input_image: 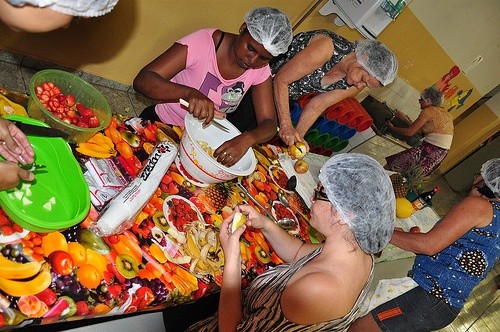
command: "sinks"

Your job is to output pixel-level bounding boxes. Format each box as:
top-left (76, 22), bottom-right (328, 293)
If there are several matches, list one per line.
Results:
top-left (360, 94), bottom-right (419, 148)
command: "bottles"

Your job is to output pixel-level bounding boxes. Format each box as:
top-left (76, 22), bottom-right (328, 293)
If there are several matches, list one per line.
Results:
top-left (411, 186), bottom-right (441, 211)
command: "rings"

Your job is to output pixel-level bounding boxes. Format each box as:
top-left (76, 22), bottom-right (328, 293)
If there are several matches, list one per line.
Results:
top-left (222, 150), bottom-right (228, 156)
top-left (229, 155), bottom-right (234, 160)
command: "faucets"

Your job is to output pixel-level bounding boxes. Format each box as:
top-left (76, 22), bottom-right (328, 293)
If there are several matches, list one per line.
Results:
top-left (383, 102), bottom-right (401, 121)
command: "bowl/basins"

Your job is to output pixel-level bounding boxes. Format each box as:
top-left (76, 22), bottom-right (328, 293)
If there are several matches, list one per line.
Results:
top-left (0, 115), bottom-right (90, 231)
top-left (27, 68), bottom-right (112, 143)
top-left (173, 111), bottom-right (257, 188)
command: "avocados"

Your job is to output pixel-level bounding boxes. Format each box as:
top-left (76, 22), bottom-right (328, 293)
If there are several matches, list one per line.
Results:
top-left (77, 227), bottom-right (112, 254)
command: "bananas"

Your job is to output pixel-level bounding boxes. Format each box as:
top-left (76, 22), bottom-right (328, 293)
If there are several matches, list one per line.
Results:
top-left (75, 132), bottom-right (117, 158)
top-left (232, 212), bottom-right (246, 233)
top-left (0, 250), bottom-right (52, 296)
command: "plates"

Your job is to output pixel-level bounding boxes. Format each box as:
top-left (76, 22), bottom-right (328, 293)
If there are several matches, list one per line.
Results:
top-left (164, 195), bottom-right (206, 237)
top-left (271, 201), bottom-right (300, 234)
top-left (269, 165), bottom-right (297, 193)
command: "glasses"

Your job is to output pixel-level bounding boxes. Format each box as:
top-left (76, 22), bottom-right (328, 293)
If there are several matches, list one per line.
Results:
top-left (313, 188), bottom-right (331, 202)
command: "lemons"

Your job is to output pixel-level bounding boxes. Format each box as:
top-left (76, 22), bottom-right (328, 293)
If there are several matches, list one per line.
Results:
top-left (93, 304), bottom-right (110, 316)
top-left (150, 244), bottom-right (168, 263)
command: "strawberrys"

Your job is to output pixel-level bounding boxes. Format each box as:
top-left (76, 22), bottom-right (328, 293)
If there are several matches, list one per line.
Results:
top-left (0, 207), bottom-right (23, 236)
top-left (272, 203), bottom-right (298, 231)
top-left (36, 81), bottom-right (99, 128)
top-left (166, 197), bottom-right (198, 232)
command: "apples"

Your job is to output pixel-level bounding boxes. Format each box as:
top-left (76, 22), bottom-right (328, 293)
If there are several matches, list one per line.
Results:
top-left (244, 166), bottom-right (277, 213)
top-left (103, 115), bottom-right (159, 178)
top-left (409, 226), bottom-right (421, 234)
top-left (294, 160), bottom-right (309, 174)
top-left (76, 301), bottom-right (90, 316)
top-left (291, 142), bottom-right (307, 158)
top-left (40, 232), bottom-right (106, 288)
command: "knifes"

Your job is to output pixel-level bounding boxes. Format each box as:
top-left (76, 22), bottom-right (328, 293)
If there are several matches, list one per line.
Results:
top-left (180, 99), bottom-right (228, 130)
top-left (20, 165), bottom-right (45, 171)
top-left (277, 127), bottom-right (302, 153)
top-left (5, 119), bottom-right (70, 137)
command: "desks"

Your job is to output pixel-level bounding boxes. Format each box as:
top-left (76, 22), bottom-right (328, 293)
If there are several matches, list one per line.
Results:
top-left (0, 87), bottom-right (443, 330)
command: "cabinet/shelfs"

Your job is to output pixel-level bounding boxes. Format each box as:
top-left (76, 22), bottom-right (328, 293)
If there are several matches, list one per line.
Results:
top-left (318, 0), bottom-right (413, 40)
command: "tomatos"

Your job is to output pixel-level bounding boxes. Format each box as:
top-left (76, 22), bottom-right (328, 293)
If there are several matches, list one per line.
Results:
top-left (19, 232), bottom-right (48, 262)
top-left (160, 175), bottom-right (178, 194)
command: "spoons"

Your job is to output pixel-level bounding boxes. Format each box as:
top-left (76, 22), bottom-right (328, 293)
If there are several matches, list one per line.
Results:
top-left (236, 183), bottom-right (296, 228)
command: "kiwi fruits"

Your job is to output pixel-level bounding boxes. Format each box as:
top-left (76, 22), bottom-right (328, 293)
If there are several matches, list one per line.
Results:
top-left (255, 246), bottom-right (271, 263)
top-left (116, 254), bottom-right (138, 278)
top-left (153, 211), bottom-right (171, 232)
top-left (234, 189), bottom-right (249, 202)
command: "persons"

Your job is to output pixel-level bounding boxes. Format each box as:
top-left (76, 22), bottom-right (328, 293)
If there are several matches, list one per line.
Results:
top-left (133, 6), bottom-right (293, 168)
top-left (346, 157), bottom-right (500, 332)
top-left (229, 29), bottom-right (399, 161)
top-left (0, 0), bottom-right (119, 192)
top-left (184, 153), bottom-right (396, 332)
top-left (382, 88), bottom-right (454, 179)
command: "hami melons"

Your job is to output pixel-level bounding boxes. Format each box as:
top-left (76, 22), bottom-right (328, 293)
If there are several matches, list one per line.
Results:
top-left (395, 198), bottom-right (414, 218)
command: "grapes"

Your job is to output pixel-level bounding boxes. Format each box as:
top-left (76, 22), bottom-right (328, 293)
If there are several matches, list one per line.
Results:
top-left (52, 270), bottom-right (169, 307)
top-left (173, 180), bottom-right (193, 200)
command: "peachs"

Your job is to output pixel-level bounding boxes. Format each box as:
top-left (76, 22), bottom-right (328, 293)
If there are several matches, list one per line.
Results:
top-left (18, 296), bottom-right (48, 318)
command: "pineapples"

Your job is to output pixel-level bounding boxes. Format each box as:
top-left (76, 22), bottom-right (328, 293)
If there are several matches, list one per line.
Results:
top-left (206, 183), bottom-right (234, 212)
top-left (390, 162), bottom-right (423, 198)
